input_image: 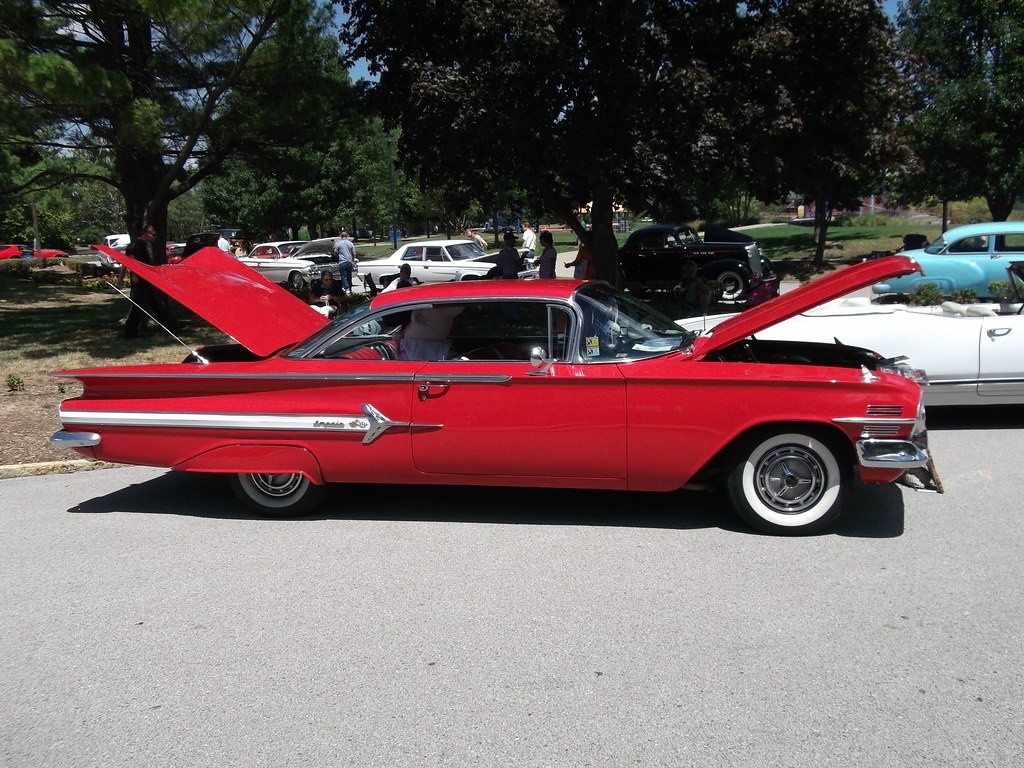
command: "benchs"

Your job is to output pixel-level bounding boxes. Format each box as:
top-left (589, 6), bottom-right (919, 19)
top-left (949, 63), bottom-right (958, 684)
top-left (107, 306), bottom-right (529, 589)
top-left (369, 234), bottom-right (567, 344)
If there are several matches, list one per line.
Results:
top-left (330, 337), bottom-right (407, 361)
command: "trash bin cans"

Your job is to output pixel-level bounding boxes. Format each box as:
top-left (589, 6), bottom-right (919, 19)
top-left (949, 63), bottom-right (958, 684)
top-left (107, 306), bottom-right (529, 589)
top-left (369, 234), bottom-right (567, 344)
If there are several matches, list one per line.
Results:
top-left (389, 229), bottom-right (402, 243)
top-left (903, 234), bottom-right (927, 251)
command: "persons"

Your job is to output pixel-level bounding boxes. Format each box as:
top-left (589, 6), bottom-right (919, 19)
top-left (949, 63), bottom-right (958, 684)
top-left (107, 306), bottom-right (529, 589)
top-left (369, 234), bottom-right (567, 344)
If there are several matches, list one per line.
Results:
top-left (278, 224), bottom-right (311, 242)
top-left (497, 232), bottom-right (527, 279)
top-left (466, 228), bottom-right (488, 250)
top-left (575, 214), bottom-right (586, 247)
top-left (516, 217), bottom-right (521, 234)
top-left (218, 232), bottom-right (255, 257)
top-left (117, 219), bottom-right (157, 339)
top-left (309, 270), bottom-right (345, 317)
top-left (521, 222), bottom-right (536, 258)
top-left (533, 231), bottom-right (558, 279)
top-left (564, 229), bottom-right (598, 279)
top-left (485, 220), bottom-right (489, 229)
top-left (45, 238), bottom-right (51, 247)
top-left (334, 232), bottom-right (357, 294)
top-left (4, 233), bottom-right (33, 247)
top-left (368, 263), bottom-right (417, 296)
top-left (382, 280), bottom-right (412, 325)
top-left (401, 227), bottom-right (406, 236)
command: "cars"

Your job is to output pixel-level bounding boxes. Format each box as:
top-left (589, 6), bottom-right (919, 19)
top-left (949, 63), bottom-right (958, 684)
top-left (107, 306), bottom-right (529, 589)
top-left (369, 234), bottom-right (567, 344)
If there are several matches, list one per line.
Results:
top-left (0, 244), bottom-right (69, 259)
top-left (616, 223), bottom-right (781, 306)
top-left (356, 240), bottom-right (540, 287)
top-left (166, 229), bottom-right (359, 295)
top-left (50, 245), bottom-right (945, 537)
top-left (672, 221), bottom-right (1024, 405)
top-left (98, 234), bottom-right (131, 269)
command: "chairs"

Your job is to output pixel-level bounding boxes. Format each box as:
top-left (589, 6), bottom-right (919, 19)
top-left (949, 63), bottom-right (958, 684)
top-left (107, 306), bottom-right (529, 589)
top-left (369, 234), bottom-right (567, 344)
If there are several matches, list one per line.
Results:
top-left (733, 275), bottom-right (781, 311)
top-left (453, 343), bottom-right (527, 360)
top-left (895, 234), bottom-right (930, 254)
top-left (364, 273), bottom-right (402, 299)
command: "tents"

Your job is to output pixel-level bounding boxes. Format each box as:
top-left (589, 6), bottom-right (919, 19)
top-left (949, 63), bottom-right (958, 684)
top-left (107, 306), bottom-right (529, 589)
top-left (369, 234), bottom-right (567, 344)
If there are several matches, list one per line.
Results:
top-left (573, 196), bottom-right (628, 233)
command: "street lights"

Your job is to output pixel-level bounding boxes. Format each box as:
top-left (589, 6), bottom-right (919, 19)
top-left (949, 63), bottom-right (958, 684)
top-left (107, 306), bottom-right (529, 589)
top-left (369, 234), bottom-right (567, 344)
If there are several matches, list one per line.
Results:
top-left (384, 131), bottom-right (397, 250)
